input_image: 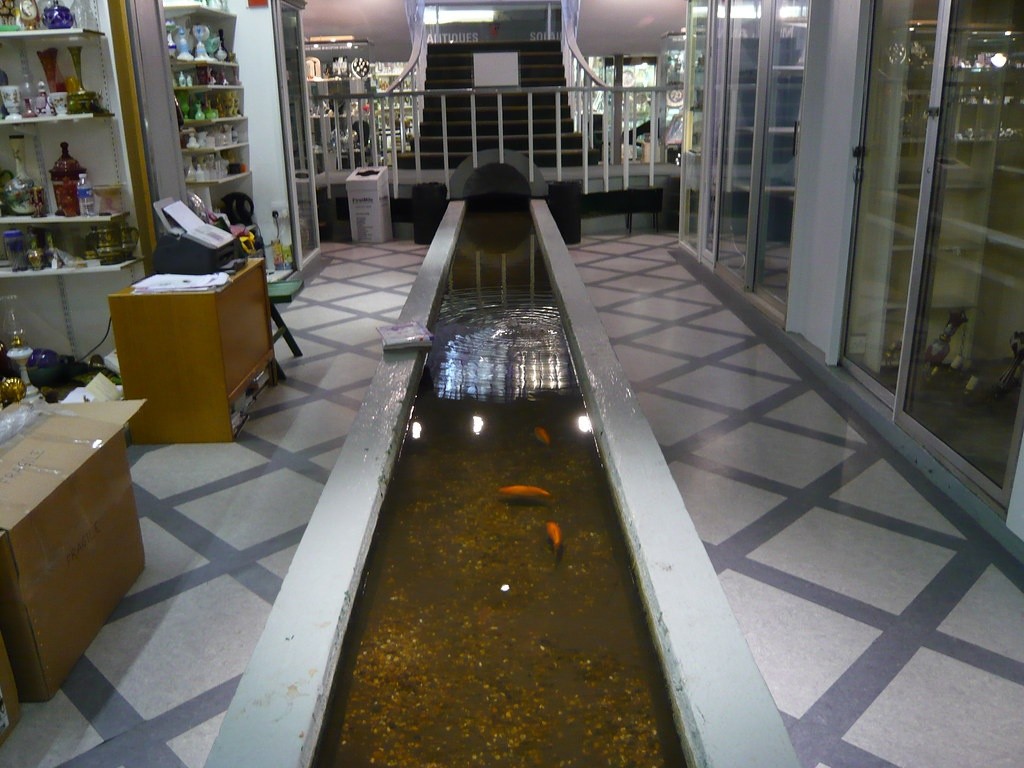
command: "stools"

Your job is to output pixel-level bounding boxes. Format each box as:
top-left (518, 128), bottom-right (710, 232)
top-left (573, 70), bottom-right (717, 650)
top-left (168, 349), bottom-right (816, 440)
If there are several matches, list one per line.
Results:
top-left (266, 280), bottom-right (304, 358)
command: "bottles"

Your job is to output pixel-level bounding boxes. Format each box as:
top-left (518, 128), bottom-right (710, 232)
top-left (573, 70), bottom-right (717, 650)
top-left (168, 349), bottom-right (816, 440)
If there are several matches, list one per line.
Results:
top-left (76, 173), bottom-right (94, 217)
top-left (179, 70), bottom-right (192, 87)
top-left (23, 98), bottom-right (37, 118)
top-left (3, 228), bottom-right (27, 272)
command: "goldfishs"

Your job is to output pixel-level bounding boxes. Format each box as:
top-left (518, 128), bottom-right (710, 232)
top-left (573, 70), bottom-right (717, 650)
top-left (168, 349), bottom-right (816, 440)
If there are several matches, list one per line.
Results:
top-left (544, 520), bottom-right (562, 554)
top-left (497, 486), bottom-right (549, 500)
top-left (534, 425), bottom-right (550, 444)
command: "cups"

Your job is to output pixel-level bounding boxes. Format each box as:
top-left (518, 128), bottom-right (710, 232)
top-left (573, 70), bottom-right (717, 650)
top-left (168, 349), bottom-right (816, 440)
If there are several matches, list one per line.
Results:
top-left (0, 85), bottom-right (23, 120)
top-left (49, 92), bottom-right (68, 117)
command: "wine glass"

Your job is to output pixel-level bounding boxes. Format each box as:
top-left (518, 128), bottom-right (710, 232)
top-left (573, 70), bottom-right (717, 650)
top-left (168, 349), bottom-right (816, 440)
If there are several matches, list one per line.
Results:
top-left (28, 186), bottom-right (47, 218)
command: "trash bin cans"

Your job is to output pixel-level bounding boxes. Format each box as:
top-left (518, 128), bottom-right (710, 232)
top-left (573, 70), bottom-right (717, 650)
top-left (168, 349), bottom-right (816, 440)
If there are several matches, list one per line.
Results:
top-left (295, 170), bottom-right (315, 249)
top-left (345, 165), bottom-right (394, 244)
top-left (411, 181), bottom-right (448, 245)
top-left (547, 180), bottom-right (581, 246)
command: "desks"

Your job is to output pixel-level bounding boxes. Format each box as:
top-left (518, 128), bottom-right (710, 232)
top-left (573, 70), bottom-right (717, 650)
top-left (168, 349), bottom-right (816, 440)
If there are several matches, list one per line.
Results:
top-left (107, 257), bottom-right (278, 444)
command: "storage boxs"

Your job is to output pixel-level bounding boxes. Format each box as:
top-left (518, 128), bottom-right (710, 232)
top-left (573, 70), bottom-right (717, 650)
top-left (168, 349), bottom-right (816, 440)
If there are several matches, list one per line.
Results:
top-left (0, 399), bottom-right (151, 702)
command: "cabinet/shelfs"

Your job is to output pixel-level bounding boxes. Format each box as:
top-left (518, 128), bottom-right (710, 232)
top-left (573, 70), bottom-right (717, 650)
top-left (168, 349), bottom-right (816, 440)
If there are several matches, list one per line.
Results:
top-left (0, 29), bottom-right (139, 274)
top-left (864, 0), bottom-right (1015, 372)
top-left (305, 40), bottom-right (370, 156)
top-left (169, 55), bottom-right (252, 184)
